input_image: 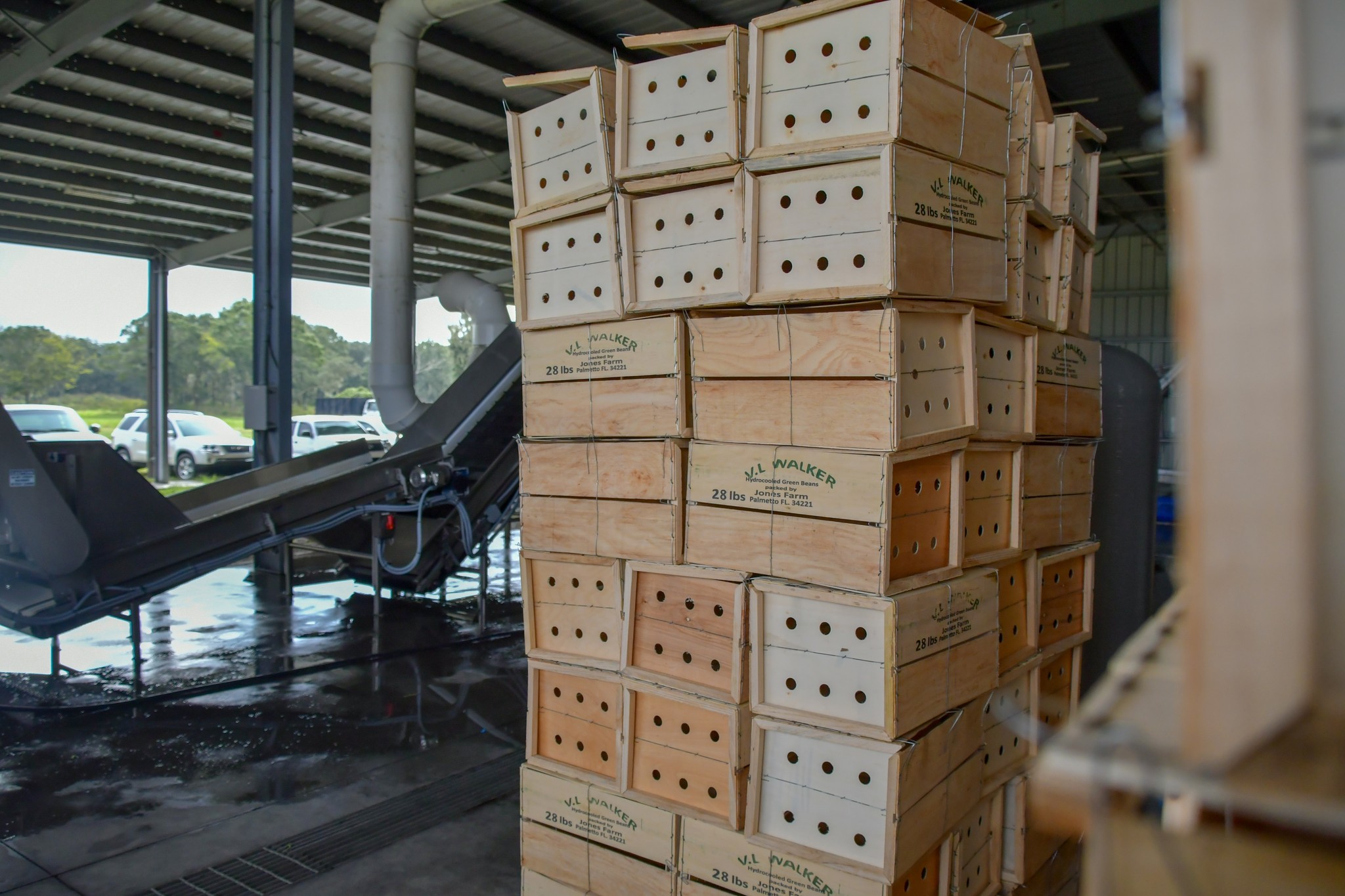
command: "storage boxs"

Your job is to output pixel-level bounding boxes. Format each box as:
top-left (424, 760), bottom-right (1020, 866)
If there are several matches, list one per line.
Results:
top-left (496, 0), bottom-right (1107, 896)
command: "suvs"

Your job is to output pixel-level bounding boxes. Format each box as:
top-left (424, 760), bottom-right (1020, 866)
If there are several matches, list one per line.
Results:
top-left (110, 409), bottom-right (255, 482)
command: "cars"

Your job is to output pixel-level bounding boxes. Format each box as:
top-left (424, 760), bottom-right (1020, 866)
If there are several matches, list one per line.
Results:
top-left (288, 400), bottom-right (400, 462)
top-left (1, 403), bottom-right (114, 447)
